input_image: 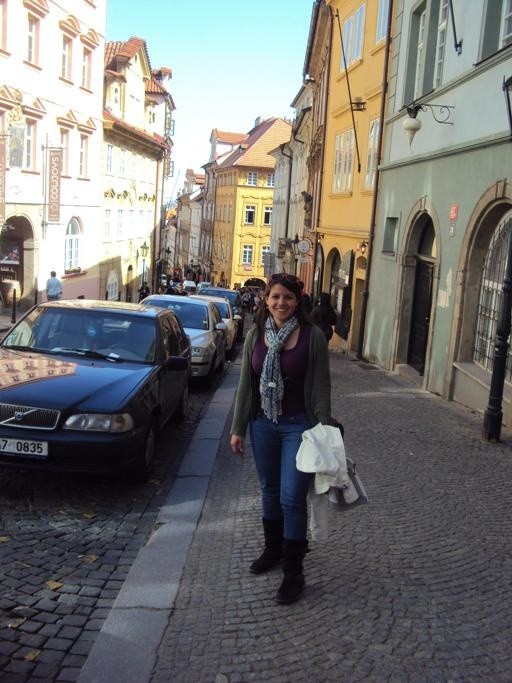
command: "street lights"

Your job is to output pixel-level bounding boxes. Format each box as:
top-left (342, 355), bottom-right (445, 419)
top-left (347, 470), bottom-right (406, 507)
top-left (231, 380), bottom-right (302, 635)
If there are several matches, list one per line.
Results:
top-left (163, 246), bottom-right (172, 279)
top-left (139, 242), bottom-right (150, 290)
top-left (477, 73), bottom-right (512, 443)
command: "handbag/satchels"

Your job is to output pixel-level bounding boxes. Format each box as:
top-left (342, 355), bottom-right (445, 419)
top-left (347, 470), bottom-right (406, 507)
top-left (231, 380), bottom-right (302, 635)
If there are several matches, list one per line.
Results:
top-left (322, 323), bottom-right (333, 340)
top-left (323, 452), bottom-right (369, 512)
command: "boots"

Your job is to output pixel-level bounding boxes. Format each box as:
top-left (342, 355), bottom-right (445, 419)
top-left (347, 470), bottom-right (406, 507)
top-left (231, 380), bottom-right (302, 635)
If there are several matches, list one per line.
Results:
top-left (249, 517), bottom-right (284, 575)
top-left (275, 534), bottom-right (309, 604)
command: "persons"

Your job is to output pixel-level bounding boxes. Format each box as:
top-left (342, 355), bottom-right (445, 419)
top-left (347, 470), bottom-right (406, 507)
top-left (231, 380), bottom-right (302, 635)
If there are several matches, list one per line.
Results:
top-left (137, 263), bottom-right (265, 315)
top-left (300, 281), bottom-right (313, 313)
top-left (46, 271), bottom-right (64, 302)
top-left (229, 272), bottom-right (336, 604)
top-left (310, 292), bottom-right (338, 346)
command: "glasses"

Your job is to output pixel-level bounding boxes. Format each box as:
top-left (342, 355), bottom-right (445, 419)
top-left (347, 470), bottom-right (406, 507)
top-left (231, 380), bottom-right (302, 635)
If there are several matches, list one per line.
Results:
top-left (268, 274), bottom-right (301, 289)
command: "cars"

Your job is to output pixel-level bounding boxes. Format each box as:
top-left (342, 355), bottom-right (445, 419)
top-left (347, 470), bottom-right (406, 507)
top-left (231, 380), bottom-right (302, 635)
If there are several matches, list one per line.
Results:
top-left (1, 294), bottom-right (192, 495)
top-left (139, 272), bottom-right (261, 385)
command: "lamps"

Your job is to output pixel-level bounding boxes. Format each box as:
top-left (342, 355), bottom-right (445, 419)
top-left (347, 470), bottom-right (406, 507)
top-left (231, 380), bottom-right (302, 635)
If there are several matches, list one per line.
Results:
top-left (360, 240), bottom-right (368, 256)
top-left (290, 234), bottom-right (313, 256)
top-left (403, 101), bottom-right (455, 149)
